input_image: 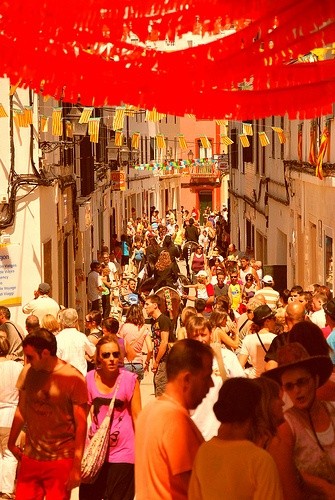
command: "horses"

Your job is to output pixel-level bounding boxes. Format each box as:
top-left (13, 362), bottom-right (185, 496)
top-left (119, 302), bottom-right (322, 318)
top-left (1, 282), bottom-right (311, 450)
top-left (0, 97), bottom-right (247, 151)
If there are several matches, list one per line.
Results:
top-left (182, 240), bottom-right (198, 282)
top-left (134, 273), bottom-right (190, 331)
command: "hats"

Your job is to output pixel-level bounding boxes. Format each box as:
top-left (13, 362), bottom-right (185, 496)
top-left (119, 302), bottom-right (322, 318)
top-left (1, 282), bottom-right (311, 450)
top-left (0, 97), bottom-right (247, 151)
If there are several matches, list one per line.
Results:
top-left (322, 297), bottom-right (335, 322)
top-left (39, 283), bottom-right (51, 294)
top-left (260, 343), bottom-right (333, 390)
top-left (214, 378), bottom-right (260, 423)
top-left (227, 255), bottom-right (238, 262)
top-left (252, 305), bottom-right (276, 322)
top-left (261, 275), bottom-right (273, 284)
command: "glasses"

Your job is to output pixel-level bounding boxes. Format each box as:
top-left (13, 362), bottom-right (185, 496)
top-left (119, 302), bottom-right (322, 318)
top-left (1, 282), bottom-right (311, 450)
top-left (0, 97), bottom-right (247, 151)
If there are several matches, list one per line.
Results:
top-left (246, 278), bottom-right (252, 282)
top-left (231, 276), bottom-right (238, 279)
top-left (269, 317), bottom-right (276, 322)
top-left (85, 319), bottom-right (91, 323)
top-left (283, 376), bottom-right (312, 391)
top-left (101, 351), bottom-right (120, 358)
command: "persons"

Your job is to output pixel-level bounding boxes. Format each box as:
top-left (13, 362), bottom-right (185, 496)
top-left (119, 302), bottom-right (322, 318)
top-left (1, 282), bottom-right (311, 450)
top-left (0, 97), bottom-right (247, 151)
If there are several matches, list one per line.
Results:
top-left (85, 205), bottom-right (335, 442)
top-left (56, 308), bottom-right (96, 378)
top-left (135, 339), bottom-right (215, 500)
top-left (26, 315), bottom-right (39, 334)
top-left (79, 336), bottom-right (141, 500)
top-left (8, 328), bottom-right (86, 500)
top-left (187, 377), bottom-right (284, 500)
top-left (40, 314), bottom-right (60, 335)
top-left (22, 283), bottom-right (60, 324)
top-left (0, 336), bottom-right (24, 499)
top-left (0, 306), bottom-right (25, 366)
top-left (260, 343), bottom-right (335, 500)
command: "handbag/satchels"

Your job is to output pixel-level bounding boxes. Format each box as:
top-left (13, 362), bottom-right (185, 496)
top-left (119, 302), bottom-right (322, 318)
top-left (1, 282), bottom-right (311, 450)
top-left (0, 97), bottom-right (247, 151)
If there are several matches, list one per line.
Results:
top-left (78, 370), bottom-right (127, 485)
